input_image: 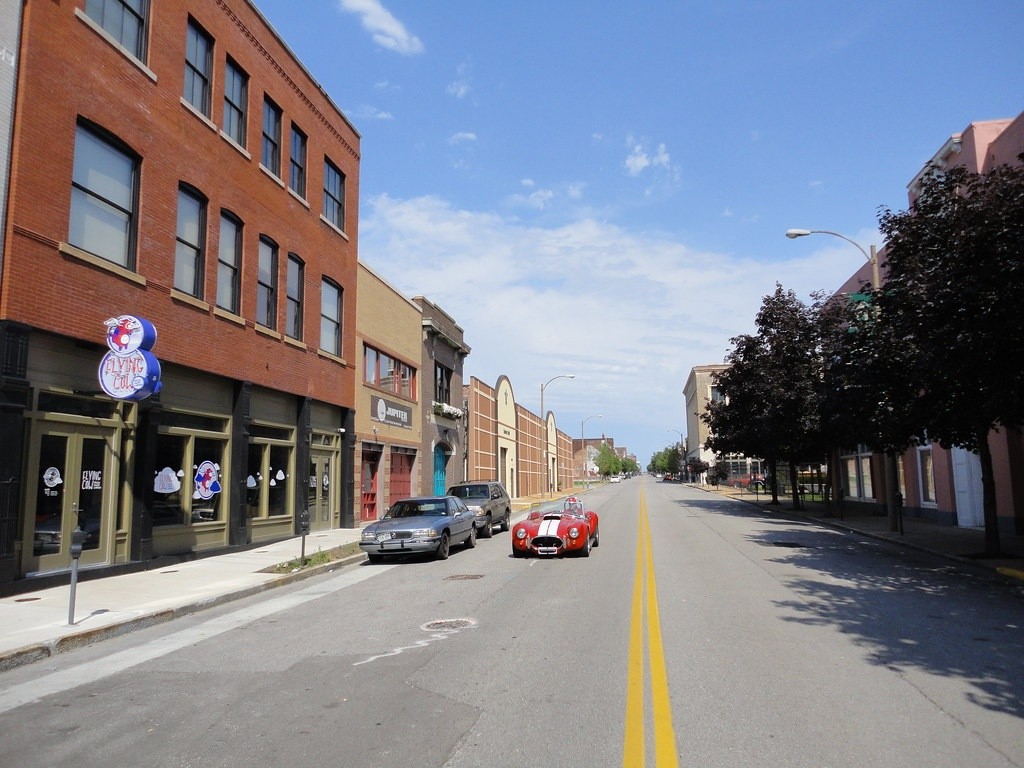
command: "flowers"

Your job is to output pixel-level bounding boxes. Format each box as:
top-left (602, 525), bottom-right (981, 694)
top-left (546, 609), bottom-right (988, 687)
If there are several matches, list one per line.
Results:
top-left (434, 402), bottom-right (462, 418)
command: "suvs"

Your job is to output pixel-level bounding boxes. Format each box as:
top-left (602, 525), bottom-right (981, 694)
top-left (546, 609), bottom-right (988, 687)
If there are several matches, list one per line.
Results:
top-left (435, 478), bottom-right (512, 538)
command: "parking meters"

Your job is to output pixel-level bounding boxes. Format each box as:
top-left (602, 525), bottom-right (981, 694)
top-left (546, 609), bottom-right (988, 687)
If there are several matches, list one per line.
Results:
top-left (838, 488), bottom-right (845, 522)
top-left (301, 510), bottom-right (311, 564)
top-left (68, 526), bottom-right (85, 627)
top-left (896, 492), bottom-right (904, 532)
top-left (550, 484), bottom-right (553, 498)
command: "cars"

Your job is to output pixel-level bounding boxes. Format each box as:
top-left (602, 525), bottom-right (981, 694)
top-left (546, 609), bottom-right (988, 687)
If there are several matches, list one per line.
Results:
top-left (610, 476), bottom-right (620, 483)
top-left (620, 473), bottom-right (632, 480)
top-left (192, 504), bottom-right (215, 523)
top-left (33, 513), bottom-right (102, 551)
top-left (650, 472), bottom-right (672, 483)
top-left (358, 495), bottom-right (476, 563)
top-left (748, 478), bottom-right (765, 489)
top-left (727, 472), bottom-right (762, 489)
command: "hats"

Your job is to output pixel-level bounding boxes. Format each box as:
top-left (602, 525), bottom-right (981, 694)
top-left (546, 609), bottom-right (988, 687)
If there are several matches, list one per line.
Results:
top-left (568, 498), bottom-right (576, 504)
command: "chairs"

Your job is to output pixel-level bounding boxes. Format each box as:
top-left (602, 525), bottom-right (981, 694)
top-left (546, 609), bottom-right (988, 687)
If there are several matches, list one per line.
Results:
top-left (564, 497), bottom-right (582, 511)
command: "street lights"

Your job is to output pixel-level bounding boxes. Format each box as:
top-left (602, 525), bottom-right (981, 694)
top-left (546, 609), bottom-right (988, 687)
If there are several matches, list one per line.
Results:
top-left (668, 430), bottom-right (684, 447)
top-left (541, 375), bottom-right (575, 498)
top-left (582, 415), bottom-right (602, 489)
top-left (662, 439), bottom-right (674, 449)
top-left (786, 228), bottom-right (902, 532)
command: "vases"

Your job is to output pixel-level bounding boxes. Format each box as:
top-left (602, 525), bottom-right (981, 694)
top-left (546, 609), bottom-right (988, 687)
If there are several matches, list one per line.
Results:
top-left (435, 412), bottom-right (461, 420)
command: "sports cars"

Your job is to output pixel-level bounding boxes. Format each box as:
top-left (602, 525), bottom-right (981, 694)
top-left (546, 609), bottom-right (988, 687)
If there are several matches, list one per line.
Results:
top-left (512, 497), bottom-right (599, 559)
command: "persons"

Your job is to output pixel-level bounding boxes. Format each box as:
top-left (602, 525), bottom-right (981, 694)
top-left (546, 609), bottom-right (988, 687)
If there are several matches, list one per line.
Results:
top-left (563, 498), bottom-right (587, 518)
